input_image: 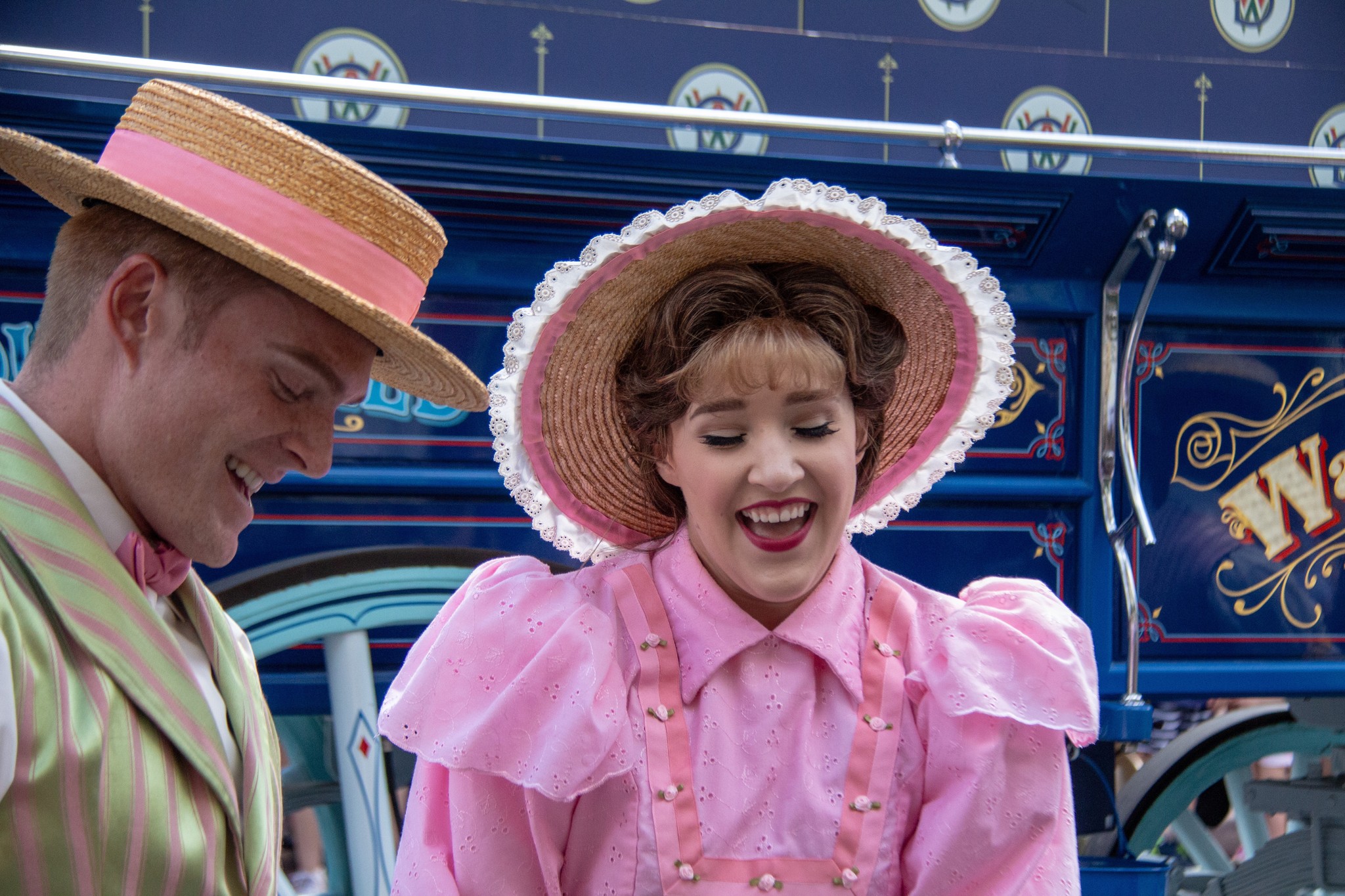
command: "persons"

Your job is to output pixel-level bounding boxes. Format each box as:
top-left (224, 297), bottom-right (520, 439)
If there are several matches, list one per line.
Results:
top-left (277, 738), bottom-right (328, 896)
top-left (375, 178), bottom-right (1098, 896)
top-left (1136, 697), bottom-right (1292, 896)
top-left (0, 80), bottom-right (489, 896)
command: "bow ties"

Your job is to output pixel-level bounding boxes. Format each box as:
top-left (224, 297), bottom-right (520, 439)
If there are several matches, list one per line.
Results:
top-left (114, 532), bottom-right (192, 598)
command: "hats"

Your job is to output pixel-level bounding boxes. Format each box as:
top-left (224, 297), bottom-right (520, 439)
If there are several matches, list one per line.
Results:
top-left (0, 77), bottom-right (489, 413)
top-left (488, 177), bottom-right (1017, 564)
